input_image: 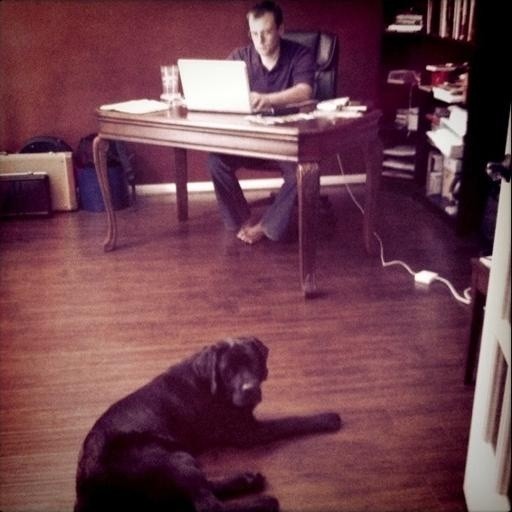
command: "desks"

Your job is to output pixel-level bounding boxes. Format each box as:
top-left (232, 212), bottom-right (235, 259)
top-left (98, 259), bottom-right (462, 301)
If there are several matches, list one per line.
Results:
top-left (92, 99), bottom-right (384, 299)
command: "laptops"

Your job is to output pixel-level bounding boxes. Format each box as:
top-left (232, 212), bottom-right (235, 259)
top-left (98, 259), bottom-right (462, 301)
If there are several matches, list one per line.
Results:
top-left (177, 58), bottom-right (264, 114)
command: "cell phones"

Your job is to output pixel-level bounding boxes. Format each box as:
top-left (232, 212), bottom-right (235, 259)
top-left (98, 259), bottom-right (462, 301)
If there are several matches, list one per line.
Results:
top-left (259, 105), bottom-right (300, 118)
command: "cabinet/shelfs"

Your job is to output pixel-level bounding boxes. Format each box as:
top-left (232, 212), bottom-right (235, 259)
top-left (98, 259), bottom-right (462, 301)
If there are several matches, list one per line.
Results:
top-left (378, 1), bottom-right (512, 238)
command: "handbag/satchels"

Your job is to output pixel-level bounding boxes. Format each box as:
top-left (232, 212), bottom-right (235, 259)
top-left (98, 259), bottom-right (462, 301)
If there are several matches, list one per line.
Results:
top-left (76, 134), bottom-right (120, 167)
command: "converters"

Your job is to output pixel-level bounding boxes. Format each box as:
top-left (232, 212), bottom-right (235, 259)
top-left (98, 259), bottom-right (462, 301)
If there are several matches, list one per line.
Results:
top-left (414, 269), bottom-right (439, 285)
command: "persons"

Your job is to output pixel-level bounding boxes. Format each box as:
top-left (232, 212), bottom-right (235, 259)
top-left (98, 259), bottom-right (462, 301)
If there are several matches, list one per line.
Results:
top-left (205, 3), bottom-right (315, 246)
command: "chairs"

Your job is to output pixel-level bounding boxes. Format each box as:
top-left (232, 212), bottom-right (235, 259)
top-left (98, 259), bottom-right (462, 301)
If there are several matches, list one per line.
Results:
top-left (242, 31), bottom-right (340, 213)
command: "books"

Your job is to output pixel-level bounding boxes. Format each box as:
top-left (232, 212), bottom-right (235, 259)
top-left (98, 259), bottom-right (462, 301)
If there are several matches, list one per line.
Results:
top-left (383, 1), bottom-right (476, 183)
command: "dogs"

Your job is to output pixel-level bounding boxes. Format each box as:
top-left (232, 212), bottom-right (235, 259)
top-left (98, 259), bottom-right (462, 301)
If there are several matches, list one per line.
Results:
top-left (72, 334), bottom-right (344, 511)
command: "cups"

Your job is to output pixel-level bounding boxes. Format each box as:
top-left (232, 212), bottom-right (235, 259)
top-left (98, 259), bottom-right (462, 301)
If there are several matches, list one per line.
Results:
top-left (160, 65), bottom-right (179, 101)
